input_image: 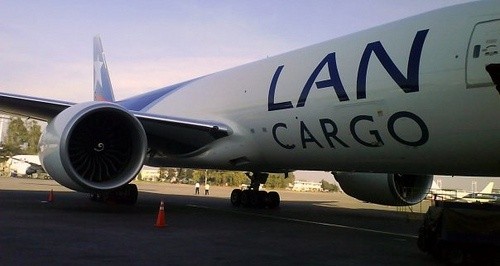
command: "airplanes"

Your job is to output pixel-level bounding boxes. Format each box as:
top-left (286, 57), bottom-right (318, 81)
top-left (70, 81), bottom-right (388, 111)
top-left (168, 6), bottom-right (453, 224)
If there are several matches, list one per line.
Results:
top-left (0, 0), bottom-right (500, 206)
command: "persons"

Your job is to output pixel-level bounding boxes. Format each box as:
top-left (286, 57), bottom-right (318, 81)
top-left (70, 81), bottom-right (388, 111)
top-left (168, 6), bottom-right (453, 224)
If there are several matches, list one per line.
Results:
top-left (194, 181), bottom-right (200, 195)
top-left (204, 182), bottom-right (210, 196)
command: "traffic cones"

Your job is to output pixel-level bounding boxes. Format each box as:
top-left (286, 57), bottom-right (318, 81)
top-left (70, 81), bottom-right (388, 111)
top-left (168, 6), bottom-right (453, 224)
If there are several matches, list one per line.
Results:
top-left (48, 188), bottom-right (54, 201)
top-left (153, 200), bottom-right (169, 227)
